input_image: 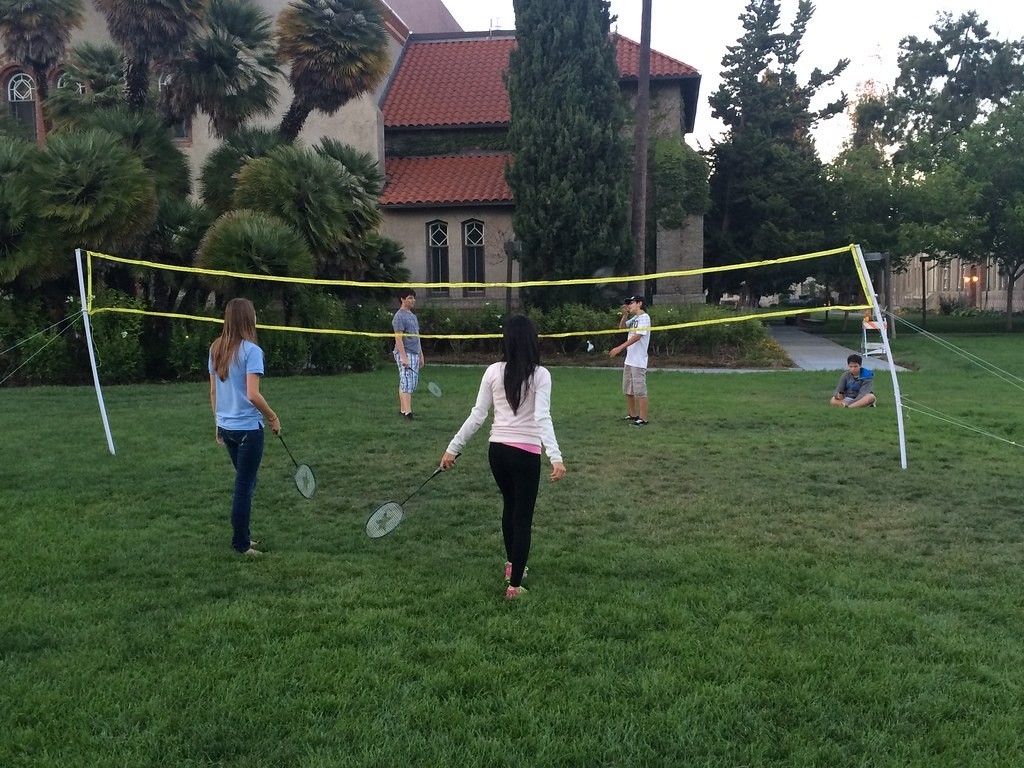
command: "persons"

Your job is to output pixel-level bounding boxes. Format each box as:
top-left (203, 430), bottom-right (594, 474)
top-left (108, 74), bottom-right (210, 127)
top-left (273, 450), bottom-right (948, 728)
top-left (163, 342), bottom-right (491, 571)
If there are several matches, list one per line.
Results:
top-left (440, 315), bottom-right (566, 601)
top-left (830, 354), bottom-right (877, 408)
top-left (208, 297), bottom-right (281, 555)
top-left (391, 288), bottom-right (424, 420)
top-left (608, 296), bottom-right (651, 426)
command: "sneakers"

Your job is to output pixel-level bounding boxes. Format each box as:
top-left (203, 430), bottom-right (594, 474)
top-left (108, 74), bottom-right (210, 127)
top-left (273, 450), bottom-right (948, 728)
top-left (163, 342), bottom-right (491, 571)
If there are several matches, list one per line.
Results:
top-left (507, 584), bottom-right (529, 598)
top-left (623, 415), bottom-right (640, 420)
top-left (632, 419), bottom-right (648, 427)
top-left (504, 561), bottom-right (529, 581)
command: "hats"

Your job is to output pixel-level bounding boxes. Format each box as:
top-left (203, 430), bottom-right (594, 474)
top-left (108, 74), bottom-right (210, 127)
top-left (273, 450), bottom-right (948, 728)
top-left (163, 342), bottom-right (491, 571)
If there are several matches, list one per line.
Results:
top-left (624, 295), bottom-right (646, 305)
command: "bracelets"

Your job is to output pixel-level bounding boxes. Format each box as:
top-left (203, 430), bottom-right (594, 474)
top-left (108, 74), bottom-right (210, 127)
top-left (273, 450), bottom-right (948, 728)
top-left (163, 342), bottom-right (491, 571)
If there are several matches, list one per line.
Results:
top-left (269, 417), bottom-right (278, 422)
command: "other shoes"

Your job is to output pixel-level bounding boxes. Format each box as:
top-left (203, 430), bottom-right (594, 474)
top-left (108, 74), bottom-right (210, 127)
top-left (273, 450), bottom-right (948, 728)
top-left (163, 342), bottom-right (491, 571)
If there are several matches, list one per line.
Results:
top-left (405, 412), bottom-right (413, 422)
top-left (867, 404), bottom-right (876, 408)
top-left (400, 411), bottom-right (403, 416)
top-left (243, 548), bottom-right (262, 555)
top-left (249, 541), bottom-right (258, 546)
